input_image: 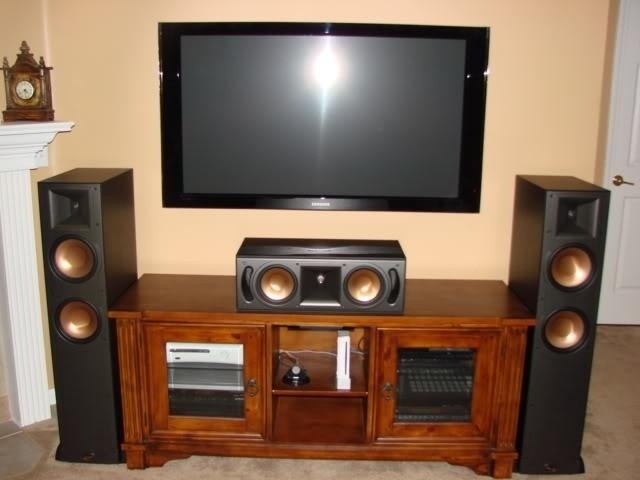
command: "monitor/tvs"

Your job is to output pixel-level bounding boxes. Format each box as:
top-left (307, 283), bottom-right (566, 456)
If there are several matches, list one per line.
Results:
top-left (157, 21), bottom-right (490, 213)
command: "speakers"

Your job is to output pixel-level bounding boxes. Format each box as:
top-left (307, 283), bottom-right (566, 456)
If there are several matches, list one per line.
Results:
top-left (508, 174), bottom-right (611, 473)
top-left (236, 237), bottom-right (407, 314)
top-left (37, 166), bottom-right (137, 463)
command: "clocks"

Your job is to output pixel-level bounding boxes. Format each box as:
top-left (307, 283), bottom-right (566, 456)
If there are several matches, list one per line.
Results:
top-left (1, 40), bottom-right (55, 122)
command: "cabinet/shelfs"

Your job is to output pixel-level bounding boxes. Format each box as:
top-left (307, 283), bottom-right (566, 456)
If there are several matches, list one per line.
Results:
top-left (108, 272), bottom-right (539, 470)
